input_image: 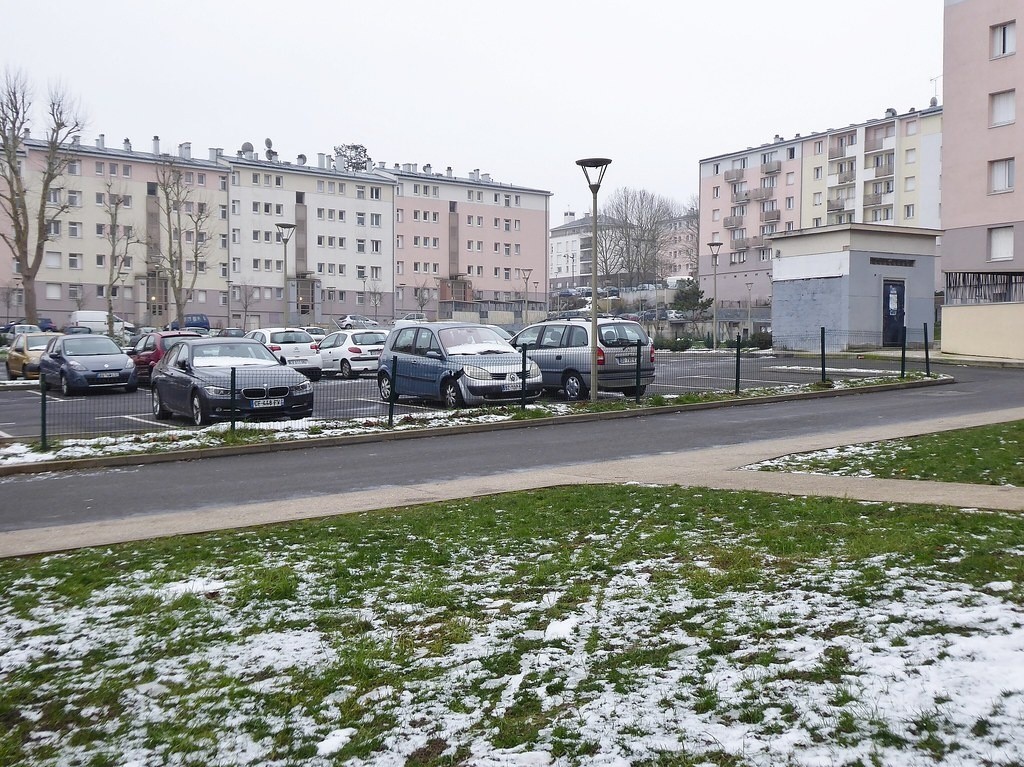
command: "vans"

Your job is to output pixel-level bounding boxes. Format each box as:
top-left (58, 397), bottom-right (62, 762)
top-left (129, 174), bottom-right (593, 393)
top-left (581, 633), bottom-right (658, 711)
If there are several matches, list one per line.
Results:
top-left (163, 313), bottom-right (211, 332)
top-left (69, 310), bottom-right (136, 336)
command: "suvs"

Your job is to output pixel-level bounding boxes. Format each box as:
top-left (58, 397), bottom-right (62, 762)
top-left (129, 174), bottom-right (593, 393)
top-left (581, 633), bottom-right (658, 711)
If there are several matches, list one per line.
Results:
top-left (507, 315), bottom-right (656, 402)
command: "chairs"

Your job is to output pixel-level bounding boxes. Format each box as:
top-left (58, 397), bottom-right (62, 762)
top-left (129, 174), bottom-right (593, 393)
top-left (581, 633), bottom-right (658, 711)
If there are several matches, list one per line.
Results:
top-left (605, 331), bottom-right (615, 340)
top-left (575, 331), bottom-right (586, 346)
top-left (546, 331), bottom-right (561, 346)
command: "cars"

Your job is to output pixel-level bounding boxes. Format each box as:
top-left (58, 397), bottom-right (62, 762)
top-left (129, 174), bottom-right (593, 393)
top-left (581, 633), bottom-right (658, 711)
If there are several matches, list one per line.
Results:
top-left (288, 326), bottom-right (327, 344)
top-left (376, 319), bottom-right (545, 412)
top-left (387, 312), bottom-right (429, 326)
top-left (37, 334), bottom-right (139, 397)
top-left (0, 317), bottom-right (246, 348)
top-left (336, 314), bottom-right (380, 330)
top-left (241, 327), bottom-right (323, 382)
top-left (149, 336), bottom-right (315, 426)
top-left (317, 328), bottom-right (390, 380)
top-left (4, 331), bottom-right (67, 381)
top-left (548, 275), bottom-right (697, 321)
top-left (126, 330), bottom-right (204, 387)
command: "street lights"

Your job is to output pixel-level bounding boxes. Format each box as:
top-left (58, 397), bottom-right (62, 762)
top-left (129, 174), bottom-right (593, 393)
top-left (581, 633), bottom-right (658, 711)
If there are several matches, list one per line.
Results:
top-left (576, 157), bottom-right (613, 405)
top-left (274, 222), bottom-right (297, 328)
top-left (520, 268), bottom-right (534, 328)
top-left (533, 281), bottom-right (540, 311)
top-left (706, 242), bottom-right (724, 351)
top-left (745, 282), bottom-right (754, 341)
top-left (555, 287), bottom-right (562, 321)
